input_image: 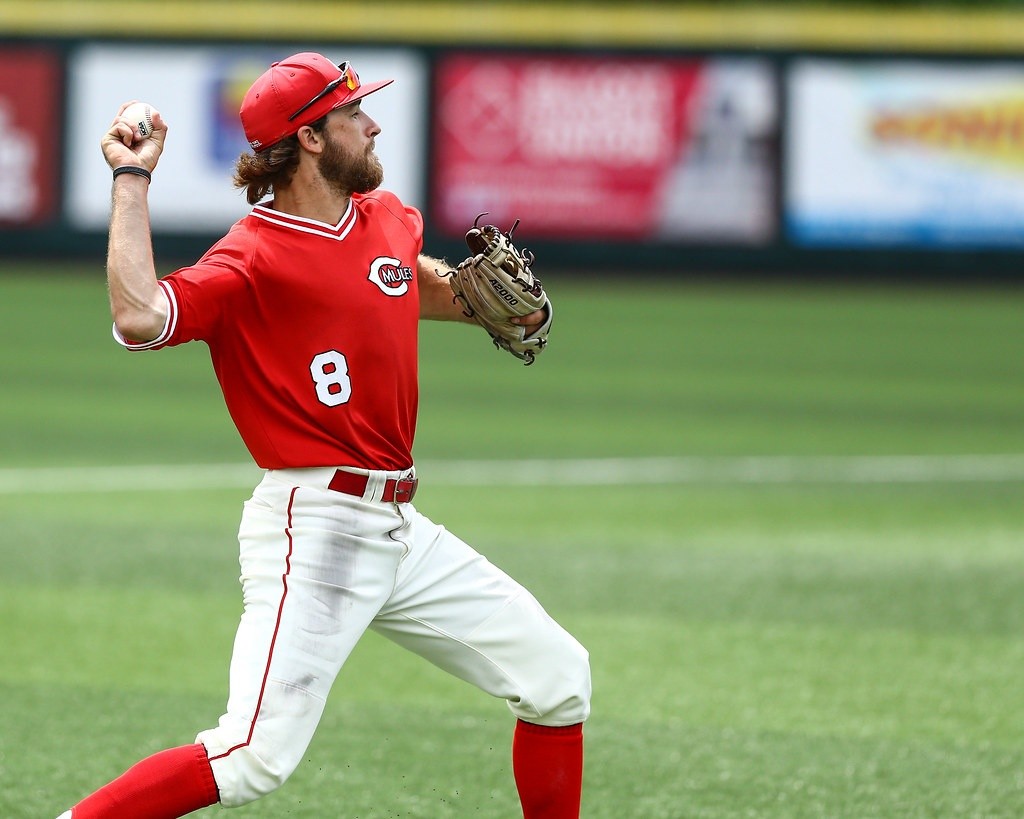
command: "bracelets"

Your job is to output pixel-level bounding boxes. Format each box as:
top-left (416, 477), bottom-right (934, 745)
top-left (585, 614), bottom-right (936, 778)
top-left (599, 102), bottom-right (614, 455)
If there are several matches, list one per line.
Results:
top-left (112, 166), bottom-right (152, 185)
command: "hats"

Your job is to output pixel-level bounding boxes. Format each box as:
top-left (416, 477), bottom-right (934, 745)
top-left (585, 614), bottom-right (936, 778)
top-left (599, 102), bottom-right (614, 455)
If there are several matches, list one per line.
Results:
top-left (239, 52), bottom-right (395, 152)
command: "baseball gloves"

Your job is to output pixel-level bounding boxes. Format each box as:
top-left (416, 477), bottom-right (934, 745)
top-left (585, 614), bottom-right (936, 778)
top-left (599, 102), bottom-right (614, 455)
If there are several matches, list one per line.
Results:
top-left (433, 211), bottom-right (555, 368)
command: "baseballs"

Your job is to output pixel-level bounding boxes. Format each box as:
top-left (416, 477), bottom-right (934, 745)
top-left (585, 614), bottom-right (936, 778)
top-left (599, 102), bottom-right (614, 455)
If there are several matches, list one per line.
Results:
top-left (120, 102), bottom-right (158, 141)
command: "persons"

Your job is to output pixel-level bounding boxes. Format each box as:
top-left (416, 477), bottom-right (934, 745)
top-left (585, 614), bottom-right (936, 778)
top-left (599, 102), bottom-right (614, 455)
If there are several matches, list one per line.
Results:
top-left (62, 51), bottom-right (591, 819)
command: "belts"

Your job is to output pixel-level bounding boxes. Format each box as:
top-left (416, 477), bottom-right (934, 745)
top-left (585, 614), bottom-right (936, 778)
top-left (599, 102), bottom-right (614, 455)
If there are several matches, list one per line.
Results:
top-left (330, 468), bottom-right (419, 503)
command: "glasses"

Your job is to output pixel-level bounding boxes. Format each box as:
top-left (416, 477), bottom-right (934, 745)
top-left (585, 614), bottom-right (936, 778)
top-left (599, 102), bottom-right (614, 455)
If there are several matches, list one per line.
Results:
top-left (289, 60), bottom-right (361, 122)
